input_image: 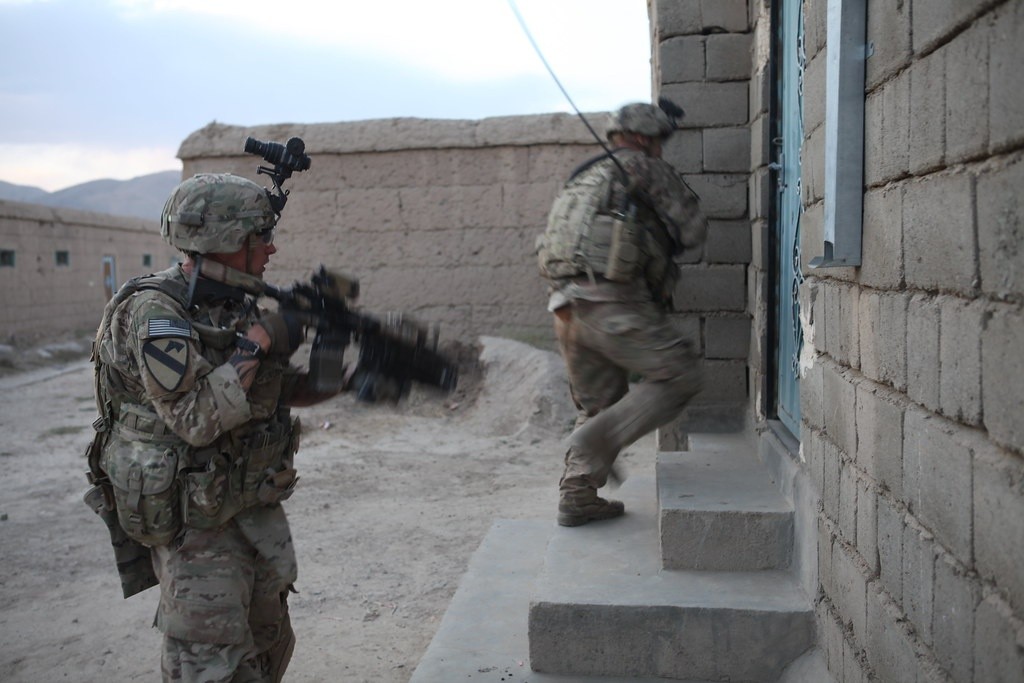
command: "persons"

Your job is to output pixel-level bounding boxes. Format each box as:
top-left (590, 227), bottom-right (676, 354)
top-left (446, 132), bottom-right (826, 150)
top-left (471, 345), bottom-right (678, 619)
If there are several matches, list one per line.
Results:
top-left (535, 103), bottom-right (707, 527)
top-left (83, 172), bottom-right (408, 683)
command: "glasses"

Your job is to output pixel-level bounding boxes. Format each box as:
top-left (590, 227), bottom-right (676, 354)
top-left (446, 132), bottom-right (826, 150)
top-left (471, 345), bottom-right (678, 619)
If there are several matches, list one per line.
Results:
top-left (251, 230), bottom-right (276, 246)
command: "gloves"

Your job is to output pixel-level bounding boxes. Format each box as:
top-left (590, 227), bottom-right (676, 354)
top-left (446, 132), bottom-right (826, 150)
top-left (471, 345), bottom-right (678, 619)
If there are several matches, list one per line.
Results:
top-left (256, 313), bottom-right (304, 360)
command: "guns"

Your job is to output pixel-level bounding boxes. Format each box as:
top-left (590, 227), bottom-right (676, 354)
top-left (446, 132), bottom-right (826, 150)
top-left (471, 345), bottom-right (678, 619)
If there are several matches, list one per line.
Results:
top-left (186, 261), bottom-right (467, 409)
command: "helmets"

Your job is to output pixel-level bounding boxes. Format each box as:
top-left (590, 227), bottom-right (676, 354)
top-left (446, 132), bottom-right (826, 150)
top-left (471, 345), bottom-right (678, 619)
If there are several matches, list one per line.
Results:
top-left (160, 174), bottom-right (274, 255)
top-left (606, 102), bottom-right (672, 138)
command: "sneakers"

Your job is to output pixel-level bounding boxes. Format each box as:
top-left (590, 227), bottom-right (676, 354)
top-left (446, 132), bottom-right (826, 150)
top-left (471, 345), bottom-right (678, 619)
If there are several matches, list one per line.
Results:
top-left (557, 496), bottom-right (624, 526)
top-left (569, 421), bottom-right (612, 487)
